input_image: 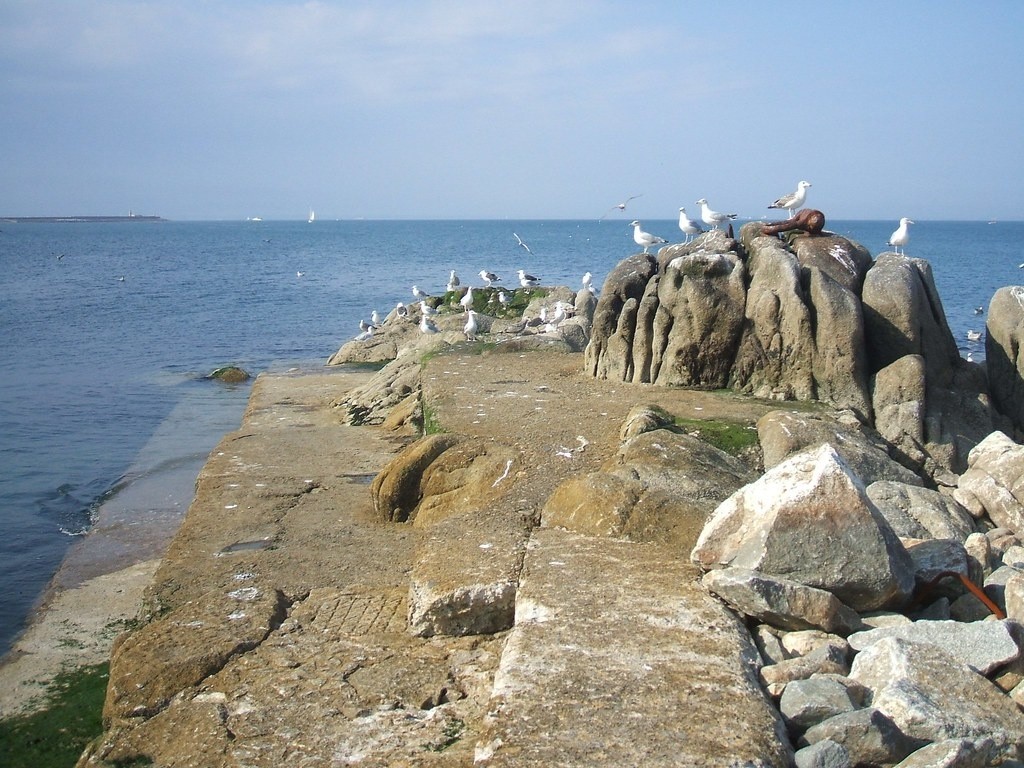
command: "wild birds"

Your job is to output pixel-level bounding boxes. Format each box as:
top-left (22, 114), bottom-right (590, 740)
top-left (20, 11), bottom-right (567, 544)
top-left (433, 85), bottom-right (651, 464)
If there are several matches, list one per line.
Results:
top-left (497, 291), bottom-right (512, 311)
top-left (601, 194), bottom-right (644, 220)
top-left (513, 233), bottom-right (533, 254)
top-left (582, 271), bottom-right (592, 289)
top-left (627, 220), bottom-right (670, 253)
top-left (766, 180), bottom-right (813, 221)
top-left (358, 269), bottom-right (544, 343)
top-left (695, 198), bottom-right (739, 229)
top-left (677, 207), bottom-right (704, 243)
top-left (974, 307), bottom-right (984, 315)
top-left (588, 283), bottom-right (601, 295)
top-left (966, 330), bottom-right (983, 339)
top-left (463, 309), bottom-right (478, 342)
top-left (884, 216), bottom-right (915, 255)
